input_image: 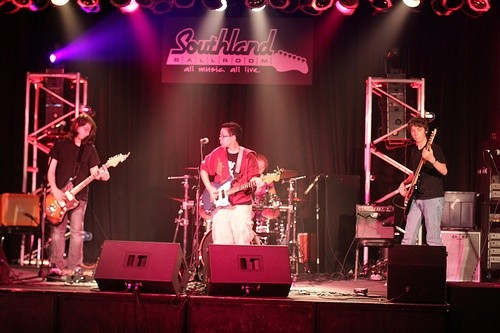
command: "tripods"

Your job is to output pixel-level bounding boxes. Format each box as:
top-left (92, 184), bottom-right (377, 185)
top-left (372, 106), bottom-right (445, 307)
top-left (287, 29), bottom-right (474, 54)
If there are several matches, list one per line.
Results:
top-left (9, 126), bottom-right (73, 285)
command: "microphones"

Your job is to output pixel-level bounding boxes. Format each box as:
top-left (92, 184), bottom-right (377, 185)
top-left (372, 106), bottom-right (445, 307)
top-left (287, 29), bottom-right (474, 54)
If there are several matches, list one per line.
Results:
top-left (303, 176), bottom-right (319, 195)
top-left (55, 121), bottom-right (67, 127)
top-left (200, 138), bottom-right (209, 144)
top-left (485, 149), bottom-right (500, 155)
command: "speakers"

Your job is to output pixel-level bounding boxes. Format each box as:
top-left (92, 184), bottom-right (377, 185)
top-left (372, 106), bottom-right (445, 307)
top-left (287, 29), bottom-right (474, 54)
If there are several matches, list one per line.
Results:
top-left (0, 193), bottom-right (40, 231)
top-left (203, 244), bottom-right (292, 297)
top-left (355, 204), bottom-right (395, 240)
top-left (295, 174), bottom-right (361, 275)
top-left (94, 240), bottom-right (189, 295)
top-left (386, 244), bottom-right (447, 304)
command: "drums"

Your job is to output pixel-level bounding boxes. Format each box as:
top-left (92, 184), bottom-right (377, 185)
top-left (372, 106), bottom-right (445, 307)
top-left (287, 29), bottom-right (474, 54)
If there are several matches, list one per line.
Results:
top-left (252, 217), bottom-right (285, 236)
top-left (199, 228), bottom-right (270, 267)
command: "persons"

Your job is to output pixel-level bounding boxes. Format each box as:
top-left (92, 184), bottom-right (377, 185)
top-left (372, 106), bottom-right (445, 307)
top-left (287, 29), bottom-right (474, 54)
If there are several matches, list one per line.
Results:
top-left (46, 114), bottom-right (110, 283)
top-left (201, 122), bottom-right (280, 246)
top-left (383, 117), bottom-right (448, 245)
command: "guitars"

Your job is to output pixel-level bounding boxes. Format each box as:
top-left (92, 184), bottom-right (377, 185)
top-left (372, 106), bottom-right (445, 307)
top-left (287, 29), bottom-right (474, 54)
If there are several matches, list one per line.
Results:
top-left (199, 173), bottom-right (280, 216)
top-left (402, 129), bottom-right (437, 218)
top-left (41, 152), bottom-right (130, 226)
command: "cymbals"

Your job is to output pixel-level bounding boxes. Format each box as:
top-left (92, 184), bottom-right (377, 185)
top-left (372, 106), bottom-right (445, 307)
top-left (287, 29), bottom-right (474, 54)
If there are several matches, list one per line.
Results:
top-left (270, 169), bottom-right (301, 180)
top-left (181, 167), bottom-right (198, 170)
top-left (168, 196), bottom-right (185, 202)
top-left (280, 197), bottom-right (312, 202)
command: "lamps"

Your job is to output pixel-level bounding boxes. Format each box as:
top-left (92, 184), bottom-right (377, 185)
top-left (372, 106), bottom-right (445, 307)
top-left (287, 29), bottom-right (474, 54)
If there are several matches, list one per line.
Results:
top-left (0, 0), bottom-right (491, 16)
top-left (23, 45), bottom-right (58, 69)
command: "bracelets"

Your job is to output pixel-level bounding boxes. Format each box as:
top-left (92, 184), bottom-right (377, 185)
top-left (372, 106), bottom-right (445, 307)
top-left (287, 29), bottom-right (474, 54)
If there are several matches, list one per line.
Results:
top-left (432, 159), bottom-right (437, 164)
top-left (100, 177), bottom-right (102, 180)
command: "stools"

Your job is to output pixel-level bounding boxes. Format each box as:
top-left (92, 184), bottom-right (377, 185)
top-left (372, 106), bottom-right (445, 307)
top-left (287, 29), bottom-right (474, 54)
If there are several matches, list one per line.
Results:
top-left (353, 240), bottom-right (393, 286)
